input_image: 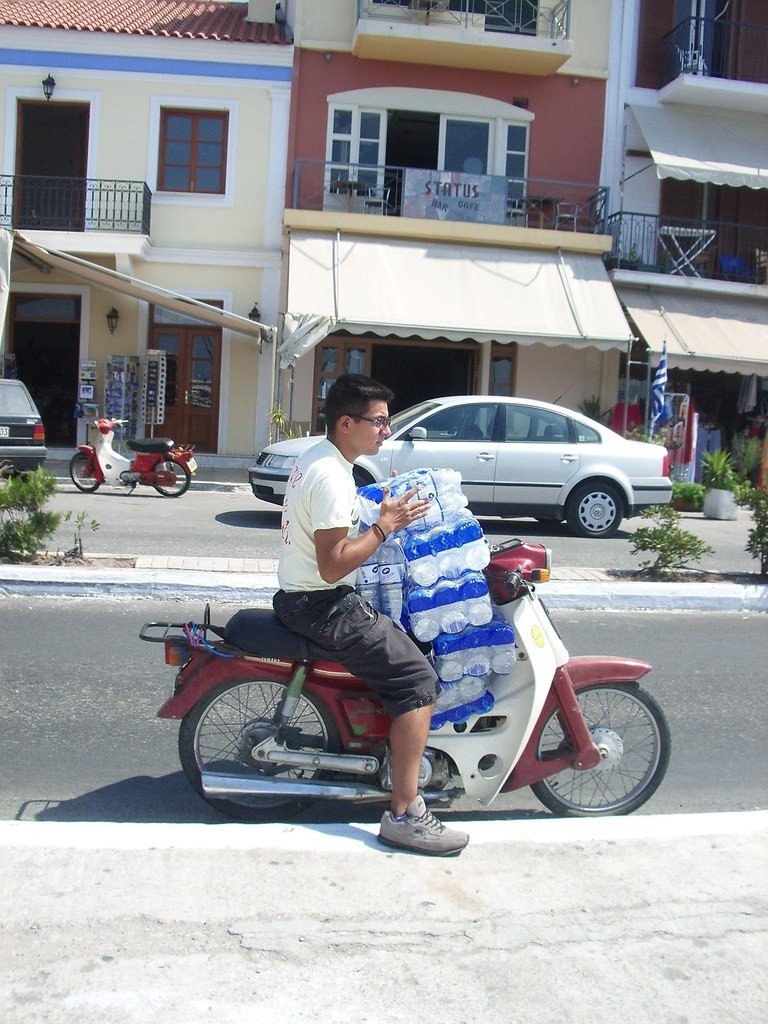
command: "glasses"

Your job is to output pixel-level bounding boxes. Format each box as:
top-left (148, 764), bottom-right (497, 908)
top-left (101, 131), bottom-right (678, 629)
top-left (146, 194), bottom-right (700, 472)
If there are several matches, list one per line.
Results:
top-left (341, 413), bottom-right (391, 429)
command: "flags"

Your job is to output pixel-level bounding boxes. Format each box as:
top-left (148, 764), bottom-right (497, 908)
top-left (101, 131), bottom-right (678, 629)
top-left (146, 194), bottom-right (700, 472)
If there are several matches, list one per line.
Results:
top-left (651, 354), bottom-right (668, 424)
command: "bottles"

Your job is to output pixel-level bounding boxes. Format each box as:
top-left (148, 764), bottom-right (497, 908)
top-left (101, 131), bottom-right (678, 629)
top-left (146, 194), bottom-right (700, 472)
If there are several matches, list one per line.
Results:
top-left (352, 466), bottom-right (517, 732)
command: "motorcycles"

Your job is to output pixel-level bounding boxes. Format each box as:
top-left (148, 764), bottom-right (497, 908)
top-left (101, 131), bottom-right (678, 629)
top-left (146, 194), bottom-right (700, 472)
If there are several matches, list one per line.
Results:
top-left (137, 540), bottom-right (672, 822)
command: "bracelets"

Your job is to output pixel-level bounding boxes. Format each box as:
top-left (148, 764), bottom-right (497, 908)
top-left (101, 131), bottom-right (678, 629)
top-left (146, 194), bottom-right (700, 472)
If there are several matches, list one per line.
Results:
top-left (372, 523), bottom-right (386, 542)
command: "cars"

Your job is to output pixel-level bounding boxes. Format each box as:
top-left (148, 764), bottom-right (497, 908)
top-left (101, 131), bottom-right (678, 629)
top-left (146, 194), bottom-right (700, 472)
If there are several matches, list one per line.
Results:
top-left (0, 378), bottom-right (48, 481)
top-left (248, 395), bottom-right (676, 542)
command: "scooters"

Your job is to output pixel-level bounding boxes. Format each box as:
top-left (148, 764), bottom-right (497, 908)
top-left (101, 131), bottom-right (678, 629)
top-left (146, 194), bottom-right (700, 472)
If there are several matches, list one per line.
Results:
top-left (69, 416), bottom-right (197, 497)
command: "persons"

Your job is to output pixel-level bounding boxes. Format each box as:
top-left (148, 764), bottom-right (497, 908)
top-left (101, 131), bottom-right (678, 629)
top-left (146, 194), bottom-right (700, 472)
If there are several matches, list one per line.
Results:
top-left (272, 373), bottom-right (469, 858)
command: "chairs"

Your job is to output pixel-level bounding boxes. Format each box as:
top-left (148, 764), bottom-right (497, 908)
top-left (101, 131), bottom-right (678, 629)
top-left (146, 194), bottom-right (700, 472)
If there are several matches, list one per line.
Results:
top-left (506, 198), bottom-right (529, 228)
top-left (363, 187), bottom-right (391, 216)
top-left (555, 201), bottom-right (590, 232)
top-left (674, 43), bottom-right (709, 76)
top-left (461, 424), bottom-right (483, 440)
top-left (755, 247), bottom-right (768, 285)
top-left (719, 254), bottom-right (756, 283)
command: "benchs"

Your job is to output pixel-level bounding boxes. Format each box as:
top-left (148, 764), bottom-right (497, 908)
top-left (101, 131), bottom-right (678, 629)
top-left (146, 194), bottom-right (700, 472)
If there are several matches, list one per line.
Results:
top-left (536, 425), bottom-right (568, 442)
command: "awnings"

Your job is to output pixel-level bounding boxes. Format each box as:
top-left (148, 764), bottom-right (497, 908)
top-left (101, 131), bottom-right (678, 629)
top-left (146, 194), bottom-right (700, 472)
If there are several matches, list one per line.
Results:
top-left (8, 231), bottom-right (277, 353)
top-left (282, 227), bottom-right (637, 353)
top-left (619, 288), bottom-right (768, 378)
top-left (631, 104), bottom-right (768, 190)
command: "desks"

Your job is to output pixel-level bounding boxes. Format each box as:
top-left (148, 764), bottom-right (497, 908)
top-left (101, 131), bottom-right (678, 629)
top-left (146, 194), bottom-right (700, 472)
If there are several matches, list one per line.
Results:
top-left (335, 181), bottom-right (369, 213)
top-left (656, 226), bottom-right (717, 278)
top-left (525, 196), bottom-right (564, 229)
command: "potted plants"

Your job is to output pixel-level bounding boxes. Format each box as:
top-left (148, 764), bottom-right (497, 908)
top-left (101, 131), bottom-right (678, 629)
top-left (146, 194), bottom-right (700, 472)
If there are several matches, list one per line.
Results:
top-left (603, 242), bottom-right (673, 274)
top-left (670, 481), bottom-right (705, 512)
top-left (699, 447), bottom-right (741, 521)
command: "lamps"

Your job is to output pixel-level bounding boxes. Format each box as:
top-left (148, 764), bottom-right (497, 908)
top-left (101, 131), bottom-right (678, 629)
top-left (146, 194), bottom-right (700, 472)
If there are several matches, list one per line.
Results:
top-left (106, 306), bottom-right (119, 335)
top-left (248, 305), bottom-right (260, 323)
top-left (41, 72), bottom-right (56, 102)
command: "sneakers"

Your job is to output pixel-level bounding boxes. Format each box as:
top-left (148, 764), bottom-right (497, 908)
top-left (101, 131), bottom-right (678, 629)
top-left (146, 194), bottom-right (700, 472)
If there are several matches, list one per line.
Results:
top-left (377, 795), bottom-right (470, 857)
